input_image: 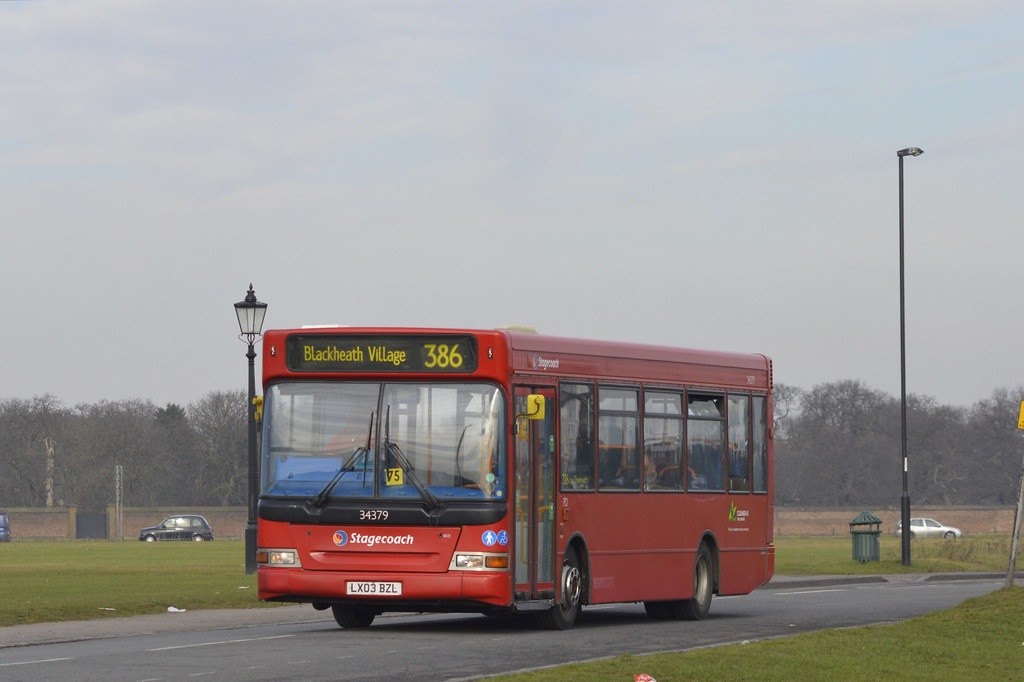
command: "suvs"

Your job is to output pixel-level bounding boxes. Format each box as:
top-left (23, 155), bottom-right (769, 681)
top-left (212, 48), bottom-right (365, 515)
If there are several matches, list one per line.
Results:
top-left (138, 514), bottom-right (215, 542)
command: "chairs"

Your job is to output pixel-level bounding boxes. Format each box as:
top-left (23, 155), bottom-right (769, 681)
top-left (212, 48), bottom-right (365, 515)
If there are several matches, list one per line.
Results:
top-left (598, 436), bottom-right (736, 491)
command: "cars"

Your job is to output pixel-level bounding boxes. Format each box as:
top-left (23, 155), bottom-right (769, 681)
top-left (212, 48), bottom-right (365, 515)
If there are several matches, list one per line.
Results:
top-left (896, 517), bottom-right (962, 539)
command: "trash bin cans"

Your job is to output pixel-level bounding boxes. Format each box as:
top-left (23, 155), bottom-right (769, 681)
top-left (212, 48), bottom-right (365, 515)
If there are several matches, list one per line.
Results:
top-left (848, 510), bottom-right (884, 563)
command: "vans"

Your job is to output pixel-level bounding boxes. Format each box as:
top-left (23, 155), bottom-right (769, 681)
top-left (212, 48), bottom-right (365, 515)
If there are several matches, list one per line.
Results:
top-left (0, 511), bottom-right (11, 542)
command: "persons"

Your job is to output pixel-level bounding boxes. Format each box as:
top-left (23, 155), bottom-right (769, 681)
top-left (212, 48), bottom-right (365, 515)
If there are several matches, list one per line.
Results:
top-left (614, 449), bottom-right (658, 487)
top-left (327, 420), bottom-right (376, 456)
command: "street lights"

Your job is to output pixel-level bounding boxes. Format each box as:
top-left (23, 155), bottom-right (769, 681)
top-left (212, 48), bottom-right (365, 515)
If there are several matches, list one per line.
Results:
top-left (232, 280), bottom-right (267, 568)
top-left (895, 146), bottom-right (925, 566)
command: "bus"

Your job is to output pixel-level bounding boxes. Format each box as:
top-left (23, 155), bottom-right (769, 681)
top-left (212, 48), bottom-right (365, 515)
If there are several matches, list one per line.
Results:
top-left (254, 325), bottom-right (776, 628)
